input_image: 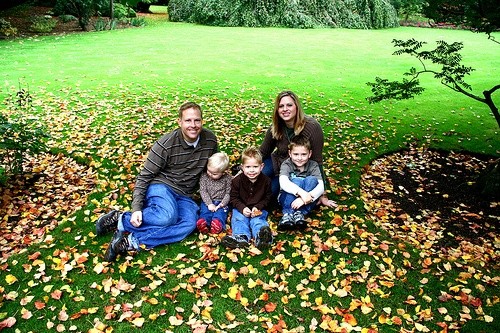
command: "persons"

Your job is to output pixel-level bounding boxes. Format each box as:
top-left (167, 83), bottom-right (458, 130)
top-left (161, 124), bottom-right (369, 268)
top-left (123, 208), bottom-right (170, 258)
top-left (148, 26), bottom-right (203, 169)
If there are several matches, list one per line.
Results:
top-left (231, 89), bottom-right (338, 209)
top-left (222, 145), bottom-right (274, 249)
top-left (196, 150), bottom-right (231, 233)
top-left (95, 102), bottom-right (218, 263)
top-left (277, 133), bottom-right (324, 229)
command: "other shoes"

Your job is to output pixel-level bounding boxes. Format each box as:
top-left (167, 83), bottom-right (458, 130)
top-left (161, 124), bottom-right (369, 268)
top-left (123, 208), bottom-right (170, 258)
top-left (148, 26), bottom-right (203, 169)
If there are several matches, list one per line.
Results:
top-left (196, 218), bottom-right (209, 234)
top-left (293, 210), bottom-right (306, 230)
top-left (277, 213), bottom-right (294, 231)
top-left (209, 218), bottom-right (223, 233)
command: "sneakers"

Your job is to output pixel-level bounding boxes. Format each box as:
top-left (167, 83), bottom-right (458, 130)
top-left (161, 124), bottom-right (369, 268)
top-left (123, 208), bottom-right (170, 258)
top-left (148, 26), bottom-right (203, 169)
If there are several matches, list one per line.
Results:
top-left (97, 209), bottom-right (121, 236)
top-left (103, 230), bottom-right (127, 262)
top-left (221, 234), bottom-right (250, 248)
top-left (254, 226), bottom-right (272, 250)
